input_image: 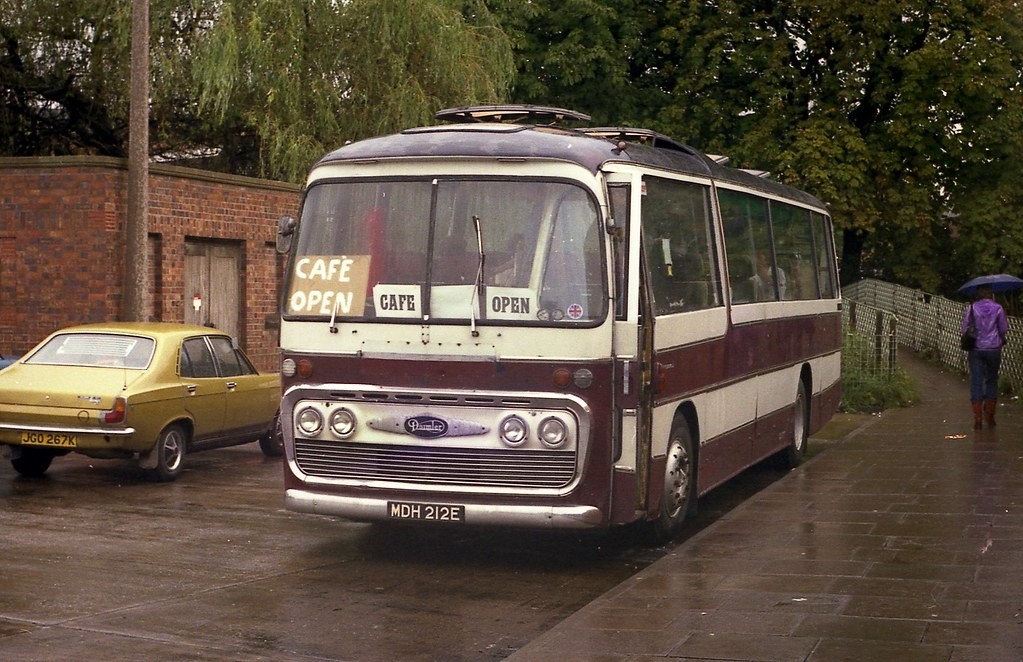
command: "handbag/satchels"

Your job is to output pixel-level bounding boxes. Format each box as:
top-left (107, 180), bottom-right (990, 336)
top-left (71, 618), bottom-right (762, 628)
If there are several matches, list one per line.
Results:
top-left (961, 303), bottom-right (976, 351)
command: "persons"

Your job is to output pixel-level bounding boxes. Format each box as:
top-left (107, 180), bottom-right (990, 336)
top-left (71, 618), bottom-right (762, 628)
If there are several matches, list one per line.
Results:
top-left (486, 178), bottom-right (568, 305)
top-left (962, 287), bottom-right (1007, 432)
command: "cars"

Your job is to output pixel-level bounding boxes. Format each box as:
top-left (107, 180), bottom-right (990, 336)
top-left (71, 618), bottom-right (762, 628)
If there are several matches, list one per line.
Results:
top-left (0, 321), bottom-right (286, 483)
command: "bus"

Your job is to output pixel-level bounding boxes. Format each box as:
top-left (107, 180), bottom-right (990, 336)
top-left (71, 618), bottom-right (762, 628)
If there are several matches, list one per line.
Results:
top-left (275, 104), bottom-right (844, 545)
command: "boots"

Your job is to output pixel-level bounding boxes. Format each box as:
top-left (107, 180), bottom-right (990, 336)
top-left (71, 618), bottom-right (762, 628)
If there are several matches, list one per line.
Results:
top-left (972, 399), bottom-right (983, 429)
top-left (984, 399), bottom-right (996, 427)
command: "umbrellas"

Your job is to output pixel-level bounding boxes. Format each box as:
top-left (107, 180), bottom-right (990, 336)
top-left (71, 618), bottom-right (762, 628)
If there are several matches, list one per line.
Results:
top-left (960, 273), bottom-right (1022, 303)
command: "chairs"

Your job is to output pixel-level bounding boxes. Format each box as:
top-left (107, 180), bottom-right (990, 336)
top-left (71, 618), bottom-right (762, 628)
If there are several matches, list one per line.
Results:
top-left (727, 253), bottom-right (755, 301)
top-left (676, 252), bottom-right (707, 308)
